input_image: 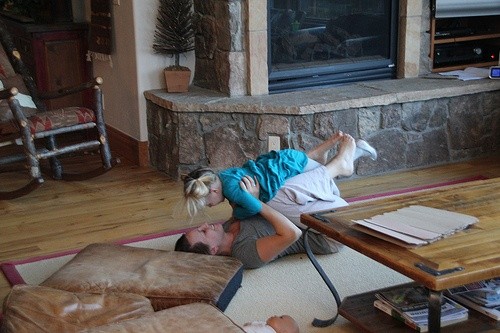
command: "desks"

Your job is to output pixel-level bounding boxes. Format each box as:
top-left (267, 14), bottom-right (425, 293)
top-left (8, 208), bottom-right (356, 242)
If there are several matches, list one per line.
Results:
top-left (300, 177), bottom-right (500, 333)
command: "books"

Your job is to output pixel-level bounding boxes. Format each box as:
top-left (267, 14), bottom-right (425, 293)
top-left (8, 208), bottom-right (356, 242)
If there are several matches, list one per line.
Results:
top-left (374, 277), bottom-right (500, 333)
top-left (438, 67), bottom-right (489, 81)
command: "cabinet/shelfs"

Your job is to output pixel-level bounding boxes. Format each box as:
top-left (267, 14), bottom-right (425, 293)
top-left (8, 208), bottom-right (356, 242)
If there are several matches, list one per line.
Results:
top-left (430, 6), bottom-right (500, 72)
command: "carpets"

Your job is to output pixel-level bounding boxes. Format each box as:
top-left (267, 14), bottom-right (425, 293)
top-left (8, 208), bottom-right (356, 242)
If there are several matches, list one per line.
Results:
top-left (1, 176), bottom-right (500, 333)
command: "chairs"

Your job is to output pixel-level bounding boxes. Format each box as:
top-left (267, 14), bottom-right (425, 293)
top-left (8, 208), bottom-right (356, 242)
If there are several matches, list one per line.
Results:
top-left (0, 27), bottom-right (120, 199)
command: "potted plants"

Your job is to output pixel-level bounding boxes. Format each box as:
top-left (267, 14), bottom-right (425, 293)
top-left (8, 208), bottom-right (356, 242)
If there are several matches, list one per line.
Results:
top-left (153, 0), bottom-right (200, 93)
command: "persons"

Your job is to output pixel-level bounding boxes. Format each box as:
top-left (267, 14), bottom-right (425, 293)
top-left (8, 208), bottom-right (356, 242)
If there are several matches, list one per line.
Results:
top-left (181, 138), bottom-right (377, 222)
top-left (175, 131), bottom-right (355, 270)
top-left (240, 314), bottom-right (299, 333)
